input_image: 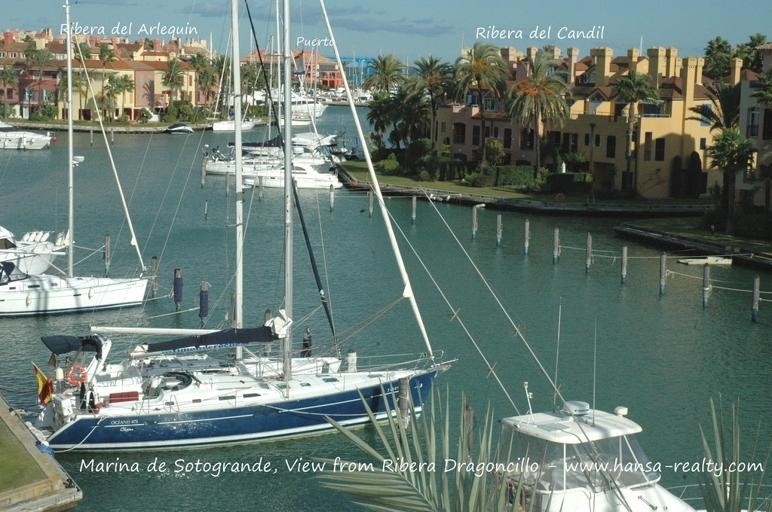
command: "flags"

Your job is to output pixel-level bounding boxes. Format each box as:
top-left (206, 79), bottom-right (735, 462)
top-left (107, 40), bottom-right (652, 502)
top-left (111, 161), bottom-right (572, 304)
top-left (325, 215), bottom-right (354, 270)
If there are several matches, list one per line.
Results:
top-left (31, 361), bottom-right (54, 407)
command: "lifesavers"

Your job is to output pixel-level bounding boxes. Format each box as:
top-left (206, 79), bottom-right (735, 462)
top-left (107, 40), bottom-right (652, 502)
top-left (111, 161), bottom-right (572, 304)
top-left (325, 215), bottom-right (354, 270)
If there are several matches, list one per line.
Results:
top-left (67, 366), bottom-right (86, 385)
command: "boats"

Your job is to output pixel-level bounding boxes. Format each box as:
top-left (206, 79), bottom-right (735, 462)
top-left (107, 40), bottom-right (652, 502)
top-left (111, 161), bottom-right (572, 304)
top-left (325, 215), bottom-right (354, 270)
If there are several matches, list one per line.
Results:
top-left (500, 401), bottom-right (693, 512)
